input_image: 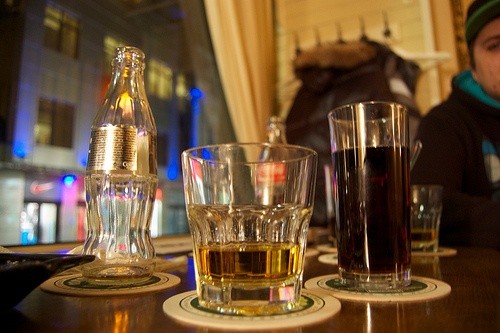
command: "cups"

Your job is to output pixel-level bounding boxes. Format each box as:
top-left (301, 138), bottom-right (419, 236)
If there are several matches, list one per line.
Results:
top-left (182, 143), bottom-right (318, 315)
top-left (411, 184), bottom-right (444, 253)
top-left (328, 101), bottom-right (411, 289)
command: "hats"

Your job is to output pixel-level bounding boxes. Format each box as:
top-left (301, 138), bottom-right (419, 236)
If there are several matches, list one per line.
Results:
top-left (465, 0), bottom-right (500, 48)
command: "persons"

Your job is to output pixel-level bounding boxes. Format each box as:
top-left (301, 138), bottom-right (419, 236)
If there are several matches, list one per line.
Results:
top-left (408, 0), bottom-right (500, 248)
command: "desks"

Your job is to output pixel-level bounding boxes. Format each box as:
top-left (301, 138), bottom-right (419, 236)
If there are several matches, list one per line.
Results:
top-left (0, 234), bottom-right (500, 333)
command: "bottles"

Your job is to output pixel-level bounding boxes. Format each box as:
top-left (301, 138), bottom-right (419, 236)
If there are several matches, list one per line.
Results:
top-left (80, 46), bottom-right (156, 284)
top-left (255, 116), bottom-right (288, 204)
